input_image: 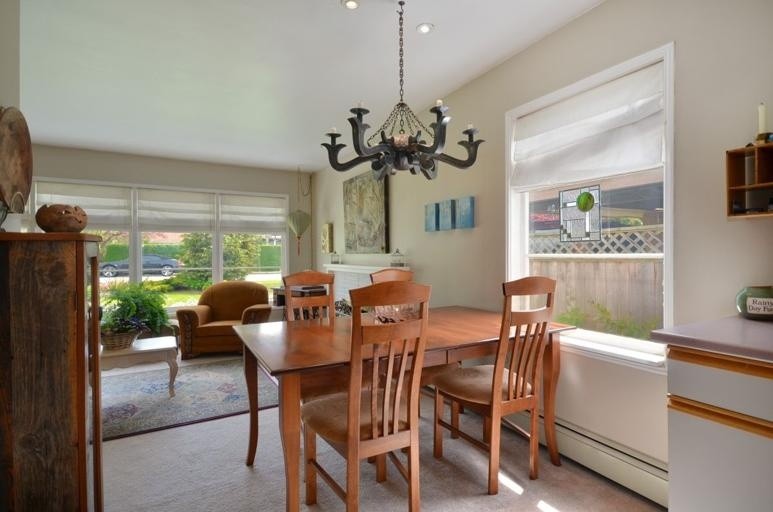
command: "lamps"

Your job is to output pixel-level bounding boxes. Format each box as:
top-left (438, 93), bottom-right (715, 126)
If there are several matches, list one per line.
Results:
top-left (284, 166), bottom-right (314, 257)
top-left (318, 1), bottom-right (486, 180)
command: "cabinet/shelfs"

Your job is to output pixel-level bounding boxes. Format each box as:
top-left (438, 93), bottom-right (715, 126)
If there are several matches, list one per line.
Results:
top-left (723, 142), bottom-right (772, 220)
top-left (651, 315), bottom-right (772, 511)
top-left (2, 232), bottom-right (107, 512)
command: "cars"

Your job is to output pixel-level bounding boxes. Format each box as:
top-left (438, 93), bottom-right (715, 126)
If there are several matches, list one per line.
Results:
top-left (97, 252), bottom-right (188, 276)
top-left (529, 206), bottom-right (560, 229)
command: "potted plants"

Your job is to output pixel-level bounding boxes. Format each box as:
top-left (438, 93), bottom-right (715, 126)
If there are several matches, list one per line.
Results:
top-left (100, 315), bottom-right (150, 353)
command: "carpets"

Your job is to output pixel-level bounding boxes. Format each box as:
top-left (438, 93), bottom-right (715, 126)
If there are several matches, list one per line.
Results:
top-left (85, 358), bottom-right (279, 448)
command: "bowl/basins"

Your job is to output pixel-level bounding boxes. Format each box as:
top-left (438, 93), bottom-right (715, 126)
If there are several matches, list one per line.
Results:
top-left (35, 203), bottom-right (88, 232)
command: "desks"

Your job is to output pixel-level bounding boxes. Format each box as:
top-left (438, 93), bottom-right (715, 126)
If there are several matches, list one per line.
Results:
top-left (88, 334), bottom-right (179, 398)
top-left (267, 285), bottom-right (326, 321)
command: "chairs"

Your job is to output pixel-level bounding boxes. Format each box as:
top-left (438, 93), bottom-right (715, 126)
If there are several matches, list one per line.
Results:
top-left (369, 265), bottom-right (461, 419)
top-left (175, 279), bottom-right (272, 361)
top-left (428, 273), bottom-right (557, 497)
top-left (282, 268), bottom-right (381, 437)
top-left (299, 280), bottom-right (432, 511)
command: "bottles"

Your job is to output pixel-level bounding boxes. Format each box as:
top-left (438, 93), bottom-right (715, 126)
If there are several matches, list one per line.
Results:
top-left (734, 285), bottom-right (772, 322)
top-left (767, 197), bottom-right (773, 212)
top-left (331, 251), bottom-right (339, 263)
top-left (390, 248), bottom-right (404, 267)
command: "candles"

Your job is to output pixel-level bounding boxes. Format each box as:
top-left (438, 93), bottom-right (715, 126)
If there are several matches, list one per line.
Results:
top-left (758, 100), bottom-right (764, 134)
